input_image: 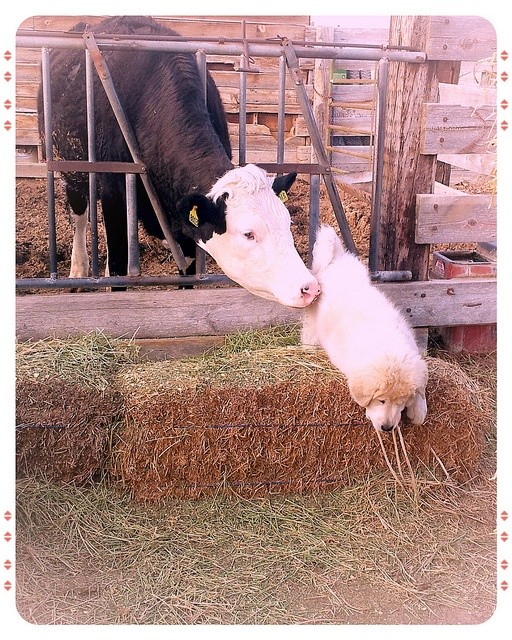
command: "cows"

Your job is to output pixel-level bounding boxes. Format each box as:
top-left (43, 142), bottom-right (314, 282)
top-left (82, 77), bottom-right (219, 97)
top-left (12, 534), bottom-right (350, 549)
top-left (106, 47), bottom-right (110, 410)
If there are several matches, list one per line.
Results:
top-left (36, 15), bottom-right (322, 310)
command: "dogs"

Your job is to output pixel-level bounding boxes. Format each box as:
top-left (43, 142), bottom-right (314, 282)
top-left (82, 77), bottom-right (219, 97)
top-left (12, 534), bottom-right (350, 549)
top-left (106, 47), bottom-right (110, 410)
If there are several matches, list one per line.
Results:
top-left (298, 224), bottom-right (429, 433)
